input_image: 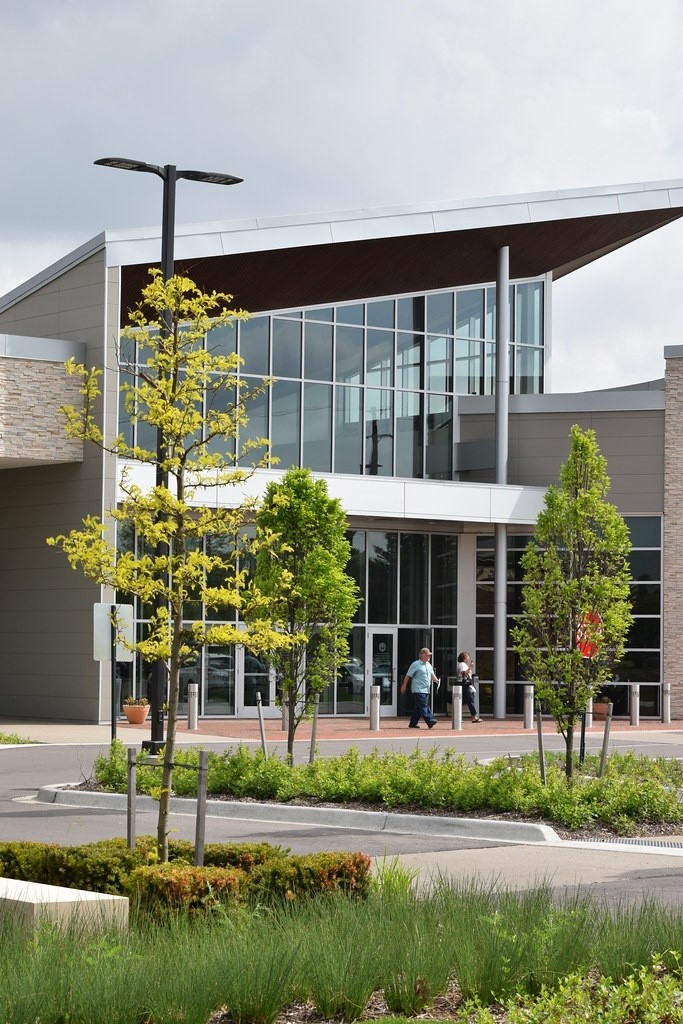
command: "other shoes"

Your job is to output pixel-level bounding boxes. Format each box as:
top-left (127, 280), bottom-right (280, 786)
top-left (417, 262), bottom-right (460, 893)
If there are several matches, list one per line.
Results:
top-left (408, 725), bottom-right (419, 728)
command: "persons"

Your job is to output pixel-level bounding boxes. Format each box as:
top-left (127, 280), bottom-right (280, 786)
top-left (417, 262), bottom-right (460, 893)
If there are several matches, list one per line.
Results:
top-left (400, 647), bottom-right (438, 729)
top-left (454, 651), bottom-right (482, 722)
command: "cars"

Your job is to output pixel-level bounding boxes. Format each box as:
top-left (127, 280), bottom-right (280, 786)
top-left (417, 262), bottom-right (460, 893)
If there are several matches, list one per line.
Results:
top-left (173, 651), bottom-right (392, 704)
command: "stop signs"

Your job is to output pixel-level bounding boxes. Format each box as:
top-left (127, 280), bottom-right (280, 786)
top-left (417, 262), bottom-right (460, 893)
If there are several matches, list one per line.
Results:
top-left (574, 607), bottom-right (604, 660)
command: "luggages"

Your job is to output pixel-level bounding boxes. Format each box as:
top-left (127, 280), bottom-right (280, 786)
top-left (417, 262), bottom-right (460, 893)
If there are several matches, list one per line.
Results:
top-left (428, 720), bottom-right (437, 729)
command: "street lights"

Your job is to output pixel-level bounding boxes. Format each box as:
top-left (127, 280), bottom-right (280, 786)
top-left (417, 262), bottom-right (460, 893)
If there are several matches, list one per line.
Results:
top-left (93, 155), bottom-right (244, 761)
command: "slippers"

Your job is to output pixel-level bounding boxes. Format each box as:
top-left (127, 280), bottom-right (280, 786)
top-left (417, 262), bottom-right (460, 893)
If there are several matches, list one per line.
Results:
top-left (472, 718), bottom-right (483, 723)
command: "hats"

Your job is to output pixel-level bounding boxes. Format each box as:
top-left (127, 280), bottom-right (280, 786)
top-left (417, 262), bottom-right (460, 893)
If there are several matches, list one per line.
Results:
top-left (421, 648), bottom-right (433, 654)
